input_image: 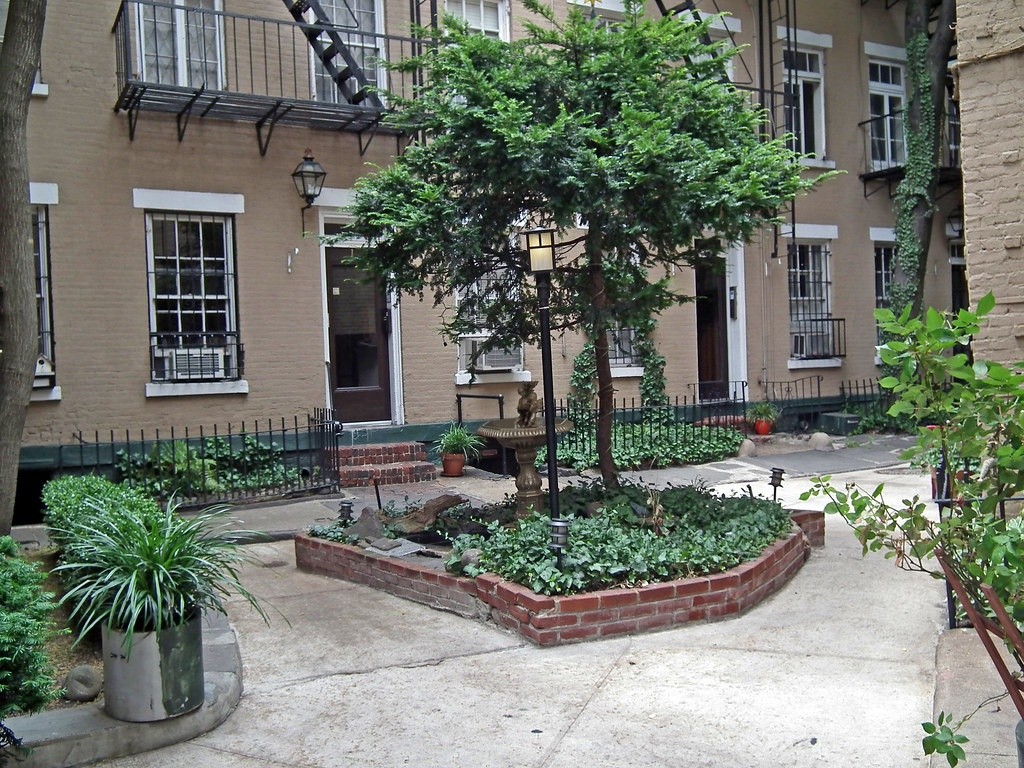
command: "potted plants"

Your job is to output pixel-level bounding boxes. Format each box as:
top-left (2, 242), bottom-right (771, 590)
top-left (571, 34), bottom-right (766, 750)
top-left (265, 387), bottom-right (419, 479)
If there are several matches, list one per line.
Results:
top-left (428, 420), bottom-right (486, 477)
top-left (34, 488), bottom-right (292, 723)
top-left (741, 401), bottom-right (784, 435)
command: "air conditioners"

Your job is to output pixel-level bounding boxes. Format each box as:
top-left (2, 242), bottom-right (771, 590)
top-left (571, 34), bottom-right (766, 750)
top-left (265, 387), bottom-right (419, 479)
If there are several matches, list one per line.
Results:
top-left (466, 337), bottom-right (524, 371)
top-left (793, 333), bottom-right (832, 358)
